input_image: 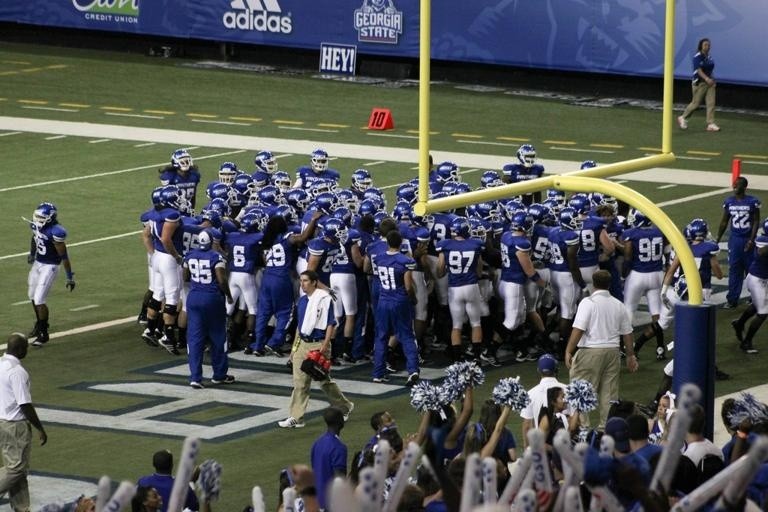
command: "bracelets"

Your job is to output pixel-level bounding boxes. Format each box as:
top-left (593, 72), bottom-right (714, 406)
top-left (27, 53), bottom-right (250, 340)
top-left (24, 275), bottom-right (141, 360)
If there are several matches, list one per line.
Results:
top-left (297, 486), bottom-right (319, 498)
top-left (705, 76), bottom-right (709, 82)
top-left (749, 240), bottom-right (753, 245)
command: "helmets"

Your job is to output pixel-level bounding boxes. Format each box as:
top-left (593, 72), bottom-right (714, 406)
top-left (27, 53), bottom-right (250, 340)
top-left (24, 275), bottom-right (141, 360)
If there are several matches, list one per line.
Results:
top-left (477, 203), bottom-right (501, 222)
top-left (288, 190), bottom-right (308, 213)
top-left (240, 213), bottom-right (262, 232)
top-left (152, 188), bottom-right (163, 211)
top-left (218, 162), bottom-right (236, 187)
top-left (543, 199), bottom-right (561, 222)
top-left (316, 193), bottom-right (338, 216)
top-left (362, 188), bottom-right (385, 211)
top-left (441, 180), bottom-right (458, 196)
top-left (271, 171), bottom-right (291, 190)
top-left (397, 184), bottom-right (419, 204)
top-left (631, 207), bottom-right (653, 230)
top-left (324, 218), bottom-right (349, 246)
top-left (351, 169), bottom-right (373, 193)
top-left (259, 187), bottom-right (282, 206)
top-left (481, 170), bottom-right (504, 188)
top-left (505, 201), bottom-right (527, 222)
top-left (449, 216), bottom-right (470, 239)
top-left (233, 174), bottom-right (256, 196)
top-left (358, 200), bottom-right (375, 217)
top-left (569, 194), bottom-right (592, 215)
top-left (510, 211), bottom-right (534, 233)
top-left (436, 162), bottom-right (462, 185)
top-left (517, 145), bottom-right (536, 168)
top-left (255, 151), bottom-right (278, 174)
top-left (337, 191), bottom-right (358, 215)
top-left (309, 181), bottom-right (329, 198)
top-left (32, 202), bottom-right (57, 231)
top-left (467, 217), bottom-right (493, 245)
top-left (210, 198), bottom-right (233, 217)
top-left (373, 212), bottom-right (391, 234)
top-left (591, 192), bottom-right (619, 216)
top-left (333, 208), bottom-right (351, 228)
top-left (528, 203), bottom-right (549, 224)
top-left (202, 210), bottom-right (222, 229)
top-left (455, 183), bottom-right (471, 195)
top-left (558, 208), bottom-right (584, 232)
top-left (159, 185), bottom-right (187, 213)
top-left (311, 149), bottom-right (328, 172)
top-left (581, 160), bottom-right (597, 170)
top-left (464, 205), bottom-right (477, 218)
top-left (411, 206), bottom-right (436, 233)
top-left (275, 205), bottom-right (299, 225)
top-left (396, 202), bottom-right (413, 220)
top-left (689, 218), bottom-right (708, 240)
top-left (249, 209), bottom-right (270, 227)
top-left (172, 149), bottom-right (194, 171)
top-left (210, 183), bottom-right (234, 202)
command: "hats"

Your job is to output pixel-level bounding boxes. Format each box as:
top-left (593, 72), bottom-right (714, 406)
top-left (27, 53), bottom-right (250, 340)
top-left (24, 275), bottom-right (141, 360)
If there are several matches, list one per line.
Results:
top-left (538, 354), bottom-right (559, 375)
top-left (198, 229), bottom-right (212, 251)
top-left (605, 417), bottom-right (630, 451)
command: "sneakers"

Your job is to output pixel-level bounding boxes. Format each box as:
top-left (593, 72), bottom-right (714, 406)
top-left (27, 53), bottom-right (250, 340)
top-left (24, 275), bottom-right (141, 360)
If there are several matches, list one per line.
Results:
top-left (154, 328), bottom-right (163, 338)
top-left (404, 373), bottom-right (419, 387)
top-left (715, 365), bottom-right (729, 380)
top-left (739, 342), bottom-right (759, 355)
top-left (253, 350), bottom-right (264, 357)
top-left (656, 345), bottom-right (666, 360)
top-left (140, 328), bottom-right (158, 346)
top-left (190, 381), bottom-right (205, 389)
top-left (28, 325), bottom-right (39, 338)
top-left (429, 342), bottom-right (447, 351)
top-left (619, 342), bottom-right (640, 361)
top-left (278, 415), bottom-right (305, 428)
top-left (732, 319), bottom-right (742, 341)
top-left (211, 375), bottom-right (234, 383)
top-left (678, 116), bottom-right (689, 129)
top-left (287, 359), bottom-right (293, 369)
top-left (330, 356), bottom-right (341, 366)
top-left (465, 344), bottom-right (476, 357)
top-left (515, 349), bottom-right (538, 363)
top-left (176, 341), bottom-right (187, 348)
top-left (479, 348), bottom-right (504, 367)
top-left (373, 378), bottom-right (390, 383)
top-left (158, 334), bottom-right (180, 356)
top-left (342, 401), bottom-right (354, 421)
top-left (418, 353), bottom-right (425, 366)
top-left (264, 344), bottom-right (285, 358)
top-left (244, 345), bottom-right (253, 354)
top-left (32, 331), bottom-right (49, 346)
top-left (635, 400), bottom-right (659, 419)
top-left (138, 314), bottom-right (148, 324)
top-left (384, 360), bottom-right (397, 373)
top-left (723, 304), bottom-right (737, 310)
top-left (350, 357), bottom-right (371, 366)
top-left (707, 122), bottom-right (721, 132)
top-left (343, 353), bottom-right (355, 363)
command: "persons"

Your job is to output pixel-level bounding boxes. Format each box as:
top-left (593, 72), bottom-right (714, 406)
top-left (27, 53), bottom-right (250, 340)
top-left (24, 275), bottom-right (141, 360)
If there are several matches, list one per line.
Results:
top-left (72, 434), bottom-right (224, 512)
top-left (135, 144), bottom-right (581, 388)
top-left (0, 332), bottom-right (47, 512)
top-left (677, 38), bottom-right (720, 132)
top-left (428, 472), bottom-right (472, 511)
top-left (617, 212), bottom-right (667, 361)
top-left (620, 225), bottom-right (694, 359)
top-left (347, 409), bottom-right (427, 511)
top-left (308, 410), bottom-right (348, 510)
top-left (277, 270), bottom-right (354, 428)
top-left (637, 275), bottom-right (688, 420)
top-left (580, 163), bottom-right (634, 299)
top-left (287, 463), bottom-right (320, 512)
top-left (27, 202), bottom-right (75, 346)
top-left (571, 389), bottom-right (768, 511)
top-left (472, 449), bottom-right (571, 511)
top-left (451, 399), bottom-right (514, 461)
top-left (731, 219), bottom-right (768, 354)
top-left (537, 387), bottom-right (569, 444)
top-left (565, 269), bottom-right (640, 437)
top-left (520, 355), bottom-right (570, 448)
top-left (428, 380), bottom-right (473, 472)
top-left (660, 219), bottom-right (729, 381)
top-left (464, 399), bottom-right (519, 463)
top-left (714, 177), bottom-right (763, 309)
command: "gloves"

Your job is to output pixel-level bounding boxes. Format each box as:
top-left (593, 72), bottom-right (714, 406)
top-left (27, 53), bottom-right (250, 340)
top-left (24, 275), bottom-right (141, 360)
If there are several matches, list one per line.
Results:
top-left (65, 272), bottom-right (75, 292)
top-left (28, 255), bottom-right (34, 263)
